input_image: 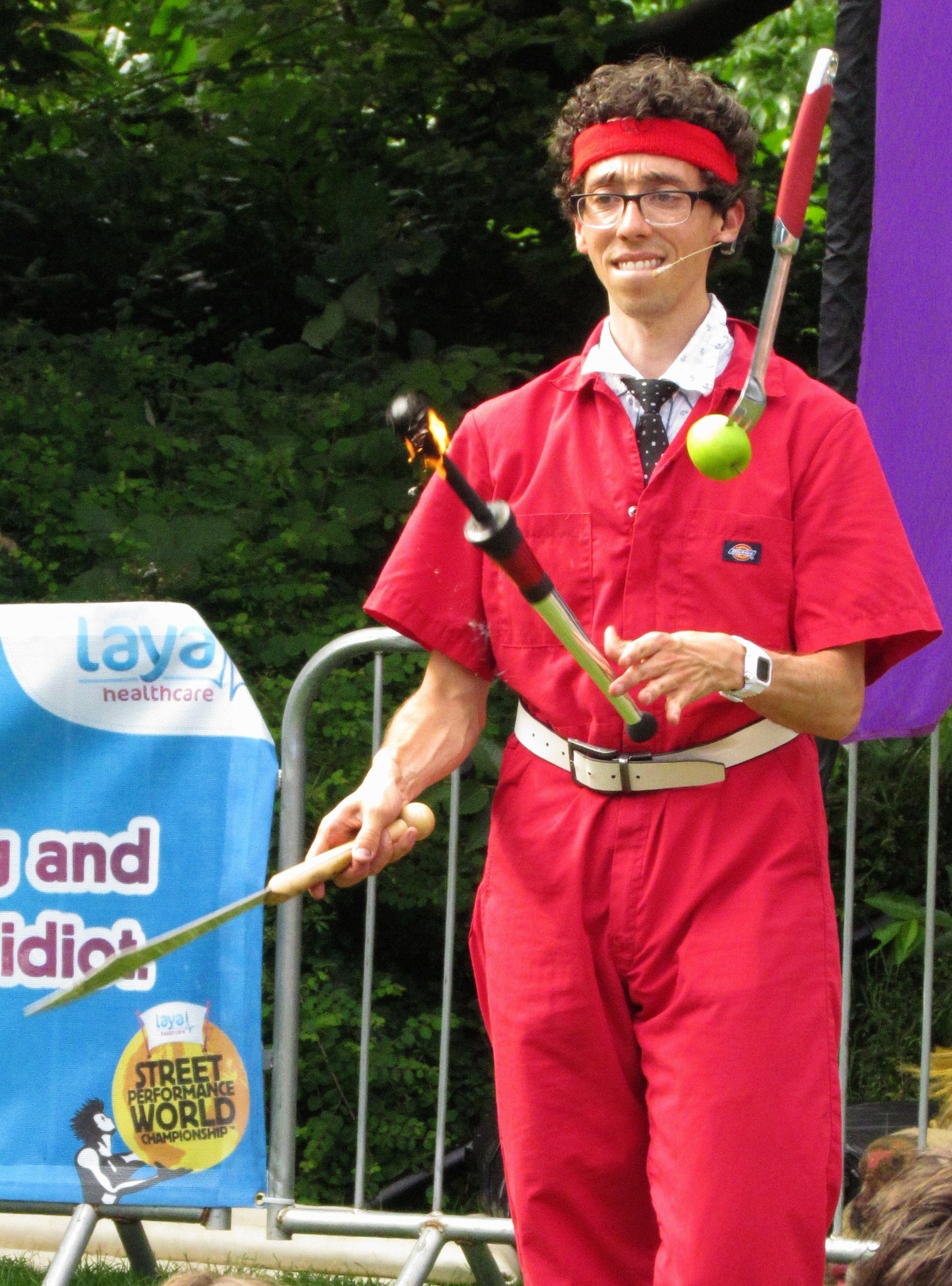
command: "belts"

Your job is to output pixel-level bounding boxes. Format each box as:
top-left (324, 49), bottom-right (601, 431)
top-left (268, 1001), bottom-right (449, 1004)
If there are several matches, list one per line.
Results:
top-left (513, 699), bottom-right (797, 794)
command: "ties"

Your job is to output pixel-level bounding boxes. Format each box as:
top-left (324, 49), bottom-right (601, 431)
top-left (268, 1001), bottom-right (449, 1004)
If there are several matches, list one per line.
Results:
top-left (621, 376), bottom-right (678, 488)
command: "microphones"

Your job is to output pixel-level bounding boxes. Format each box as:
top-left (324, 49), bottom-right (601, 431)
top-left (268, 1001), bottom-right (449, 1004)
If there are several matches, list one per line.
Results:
top-left (652, 241), bottom-right (723, 279)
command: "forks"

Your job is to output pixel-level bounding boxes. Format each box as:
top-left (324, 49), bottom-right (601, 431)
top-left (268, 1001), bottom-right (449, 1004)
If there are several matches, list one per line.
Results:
top-left (729, 45), bottom-right (841, 431)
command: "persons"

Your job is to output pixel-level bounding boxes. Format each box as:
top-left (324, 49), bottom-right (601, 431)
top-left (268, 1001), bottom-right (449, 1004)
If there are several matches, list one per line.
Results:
top-left (306, 55), bottom-right (944, 1286)
top-left (855, 1154), bottom-right (952, 1286)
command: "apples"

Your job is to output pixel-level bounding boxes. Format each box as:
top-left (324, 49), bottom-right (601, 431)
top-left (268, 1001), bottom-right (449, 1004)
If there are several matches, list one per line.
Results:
top-left (687, 414), bottom-right (751, 480)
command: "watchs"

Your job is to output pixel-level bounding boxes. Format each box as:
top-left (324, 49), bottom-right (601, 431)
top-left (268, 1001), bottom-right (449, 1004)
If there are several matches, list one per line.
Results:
top-left (719, 635), bottom-right (772, 702)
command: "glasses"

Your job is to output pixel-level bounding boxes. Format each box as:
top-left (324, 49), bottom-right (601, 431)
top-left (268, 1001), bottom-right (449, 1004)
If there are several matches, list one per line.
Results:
top-left (569, 190), bottom-right (712, 229)
